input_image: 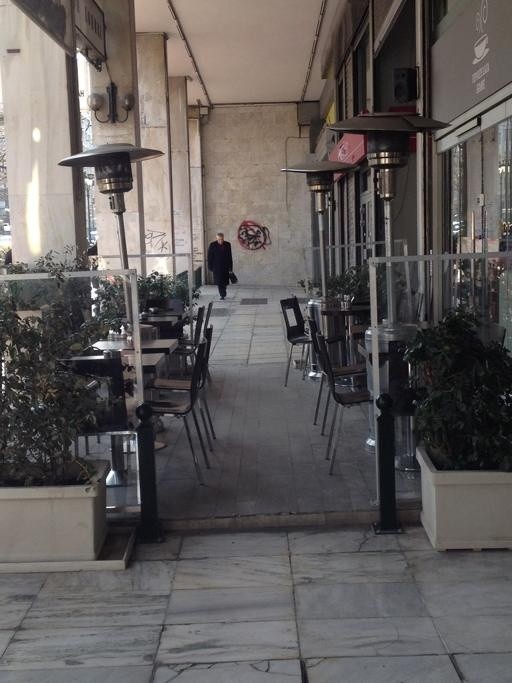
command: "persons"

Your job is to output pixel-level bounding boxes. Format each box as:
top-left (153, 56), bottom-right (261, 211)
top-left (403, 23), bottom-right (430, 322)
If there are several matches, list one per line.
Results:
top-left (207, 232), bottom-right (232, 299)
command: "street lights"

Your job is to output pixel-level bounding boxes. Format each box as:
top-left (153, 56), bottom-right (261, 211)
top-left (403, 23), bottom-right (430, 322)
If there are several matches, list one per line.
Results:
top-left (85, 177), bottom-right (92, 244)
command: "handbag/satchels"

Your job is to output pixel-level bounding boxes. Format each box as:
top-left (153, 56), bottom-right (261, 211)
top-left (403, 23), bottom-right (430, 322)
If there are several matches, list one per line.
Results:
top-left (229, 270), bottom-right (239, 284)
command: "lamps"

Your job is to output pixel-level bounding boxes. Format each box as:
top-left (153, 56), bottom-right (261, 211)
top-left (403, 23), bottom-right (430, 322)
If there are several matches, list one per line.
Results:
top-left (86, 78), bottom-right (136, 124)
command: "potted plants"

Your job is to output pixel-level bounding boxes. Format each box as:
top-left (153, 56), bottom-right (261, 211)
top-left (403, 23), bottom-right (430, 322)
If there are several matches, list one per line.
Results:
top-left (1, 268), bottom-right (112, 563)
top-left (400, 304), bottom-right (512, 551)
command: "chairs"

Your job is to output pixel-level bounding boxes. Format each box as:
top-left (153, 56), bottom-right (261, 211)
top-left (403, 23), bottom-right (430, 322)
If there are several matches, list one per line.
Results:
top-left (277, 294), bottom-right (512, 475)
top-left (129, 301), bottom-right (219, 487)
top-left (55, 353), bottom-right (133, 489)
